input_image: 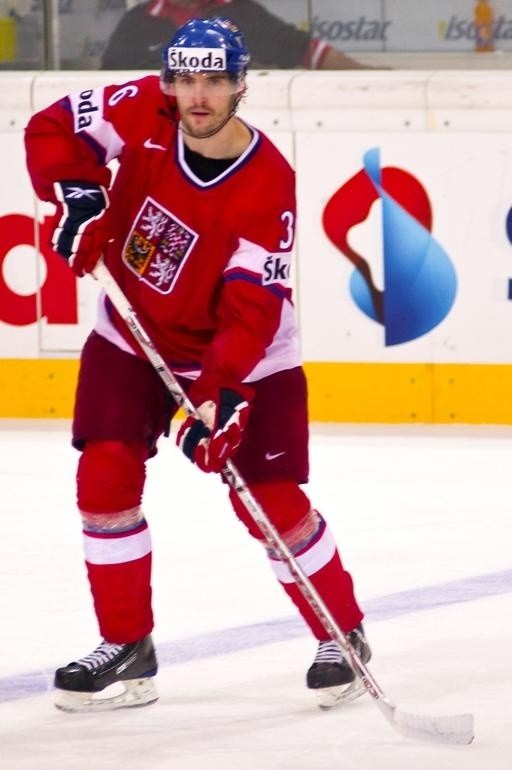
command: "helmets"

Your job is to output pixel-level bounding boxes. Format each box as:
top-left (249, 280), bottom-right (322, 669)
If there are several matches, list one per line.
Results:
top-left (159, 15), bottom-right (250, 85)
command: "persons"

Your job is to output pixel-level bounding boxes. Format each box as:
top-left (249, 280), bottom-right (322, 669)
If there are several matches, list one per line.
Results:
top-left (24, 18), bottom-right (372, 692)
top-left (101, 0), bottom-right (367, 70)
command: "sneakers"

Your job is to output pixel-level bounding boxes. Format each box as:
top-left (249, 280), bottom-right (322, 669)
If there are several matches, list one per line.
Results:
top-left (307, 623), bottom-right (371, 689)
top-left (55, 633), bottom-right (157, 692)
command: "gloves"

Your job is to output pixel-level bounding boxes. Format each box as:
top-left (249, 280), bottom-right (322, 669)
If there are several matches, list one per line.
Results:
top-left (48, 180), bottom-right (111, 277)
top-left (165, 374), bottom-right (255, 472)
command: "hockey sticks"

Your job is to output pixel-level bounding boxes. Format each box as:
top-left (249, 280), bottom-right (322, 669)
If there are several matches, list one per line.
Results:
top-left (89, 260), bottom-right (475, 746)
